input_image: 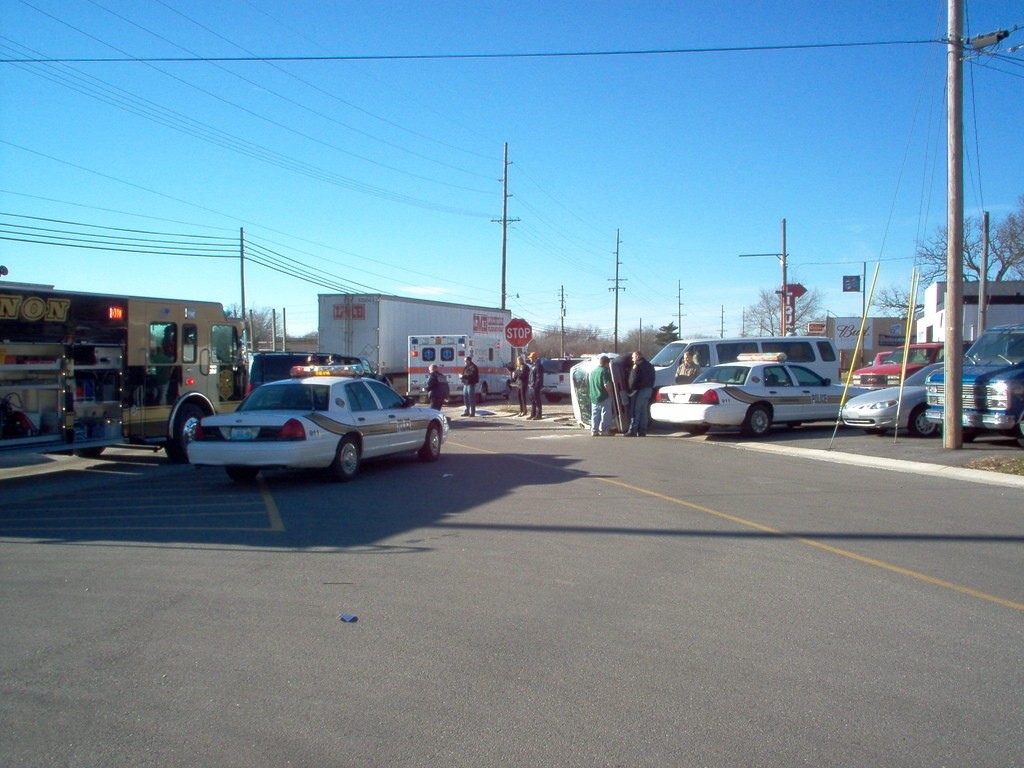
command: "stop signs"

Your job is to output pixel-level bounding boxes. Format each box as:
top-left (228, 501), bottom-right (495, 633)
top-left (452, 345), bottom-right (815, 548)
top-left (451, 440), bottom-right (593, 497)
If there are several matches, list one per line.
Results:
top-left (504, 318), bottom-right (533, 348)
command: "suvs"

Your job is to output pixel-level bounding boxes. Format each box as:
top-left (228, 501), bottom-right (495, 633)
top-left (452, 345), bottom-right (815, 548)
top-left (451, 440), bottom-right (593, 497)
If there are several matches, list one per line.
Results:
top-left (248, 351), bottom-right (386, 396)
top-left (925, 324), bottom-right (1024, 450)
top-left (853, 340), bottom-right (976, 388)
top-left (539, 355), bottom-right (588, 403)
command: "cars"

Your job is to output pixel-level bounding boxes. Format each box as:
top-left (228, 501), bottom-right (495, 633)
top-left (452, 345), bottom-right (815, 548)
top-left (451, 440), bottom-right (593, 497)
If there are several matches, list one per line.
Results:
top-left (185, 365), bottom-right (449, 486)
top-left (871, 350), bottom-right (892, 366)
top-left (841, 360), bottom-right (945, 438)
top-left (651, 351), bottom-right (870, 440)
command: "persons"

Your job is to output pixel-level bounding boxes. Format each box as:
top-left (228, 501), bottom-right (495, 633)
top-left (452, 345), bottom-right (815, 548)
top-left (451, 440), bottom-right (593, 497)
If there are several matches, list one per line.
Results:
top-left (422, 364), bottom-right (450, 410)
top-left (623, 351), bottom-right (656, 437)
top-left (512, 356), bottom-right (531, 417)
top-left (588, 356), bottom-right (615, 436)
top-left (459, 356), bottom-right (479, 416)
top-left (675, 352), bottom-right (701, 384)
top-left (296, 354), bottom-right (340, 368)
top-left (525, 352), bottom-right (544, 420)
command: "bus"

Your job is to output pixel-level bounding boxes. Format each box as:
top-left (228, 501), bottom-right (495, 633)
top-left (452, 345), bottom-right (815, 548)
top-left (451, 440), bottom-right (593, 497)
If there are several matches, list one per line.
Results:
top-left (0, 280), bottom-right (245, 464)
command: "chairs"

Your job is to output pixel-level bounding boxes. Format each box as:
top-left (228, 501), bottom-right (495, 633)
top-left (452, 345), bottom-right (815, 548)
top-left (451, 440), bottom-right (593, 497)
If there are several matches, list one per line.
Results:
top-left (767, 374), bottom-right (778, 386)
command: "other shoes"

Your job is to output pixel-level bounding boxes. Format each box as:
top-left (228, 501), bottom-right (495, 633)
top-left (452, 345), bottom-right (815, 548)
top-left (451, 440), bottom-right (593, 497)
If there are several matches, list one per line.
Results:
top-left (622, 432), bottom-right (637, 437)
top-left (517, 412), bottom-right (522, 417)
top-left (533, 416), bottom-right (542, 420)
top-left (636, 432), bottom-right (646, 437)
top-left (468, 414), bottom-right (474, 417)
top-left (460, 413), bottom-right (469, 417)
top-left (526, 415), bottom-right (534, 420)
top-left (598, 430), bottom-right (615, 436)
top-left (591, 431), bottom-right (598, 436)
top-left (521, 412), bottom-right (525, 416)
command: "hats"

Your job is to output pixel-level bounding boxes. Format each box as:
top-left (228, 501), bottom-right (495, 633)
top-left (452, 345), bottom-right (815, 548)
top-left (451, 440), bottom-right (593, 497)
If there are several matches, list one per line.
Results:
top-left (527, 352), bottom-right (536, 358)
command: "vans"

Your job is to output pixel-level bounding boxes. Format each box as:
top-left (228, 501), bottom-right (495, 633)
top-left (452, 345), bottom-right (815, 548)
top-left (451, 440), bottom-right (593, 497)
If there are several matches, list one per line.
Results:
top-left (647, 334), bottom-right (842, 400)
top-left (406, 335), bottom-right (511, 405)
